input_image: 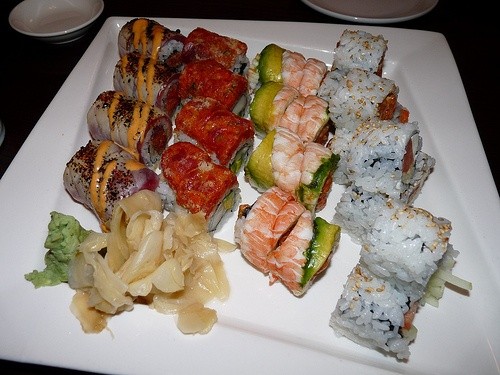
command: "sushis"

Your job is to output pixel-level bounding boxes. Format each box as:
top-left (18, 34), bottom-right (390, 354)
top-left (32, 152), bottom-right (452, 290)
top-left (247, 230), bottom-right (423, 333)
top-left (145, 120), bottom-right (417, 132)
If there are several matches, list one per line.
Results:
top-left (245, 43), bottom-right (327, 97)
top-left (117, 17), bottom-right (190, 67)
top-left (159, 141), bottom-right (243, 233)
top-left (360, 203), bottom-right (474, 303)
top-left (88, 90), bottom-right (175, 165)
top-left (64, 138), bottom-right (160, 233)
top-left (243, 127), bottom-right (339, 212)
top-left (335, 188), bottom-right (396, 240)
top-left (250, 80), bottom-right (331, 146)
top-left (172, 96), bottom-right (256, 173)
top-left (112, 50), bottom-right (182, 118)
top-left (330, 258), bottom-right (419, 360)
top-left (185, 26), bottom-right (248, 75)
top-left (329, 119), bottom-right (433, 204)
top-left (179, 59), bottom-right (251, 116)
top-left (330, 29), bottom-right (388, 76)
top-left (233, 186), bottom-right (340, 296)
top-left (316, 68), bottom-right (410, 129)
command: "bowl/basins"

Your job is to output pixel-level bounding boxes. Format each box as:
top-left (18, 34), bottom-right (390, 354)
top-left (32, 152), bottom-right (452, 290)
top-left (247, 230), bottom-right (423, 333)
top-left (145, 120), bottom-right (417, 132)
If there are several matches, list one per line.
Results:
top-left (9, 0), bottom-right (104, 45)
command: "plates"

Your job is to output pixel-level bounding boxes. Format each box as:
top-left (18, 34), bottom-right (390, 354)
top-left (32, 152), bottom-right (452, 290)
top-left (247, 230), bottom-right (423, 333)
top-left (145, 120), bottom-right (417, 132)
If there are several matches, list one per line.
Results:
top-left (303, 0), bottom-right (439, 24)
top-left (1, 17), bottom-right (500, 375)
